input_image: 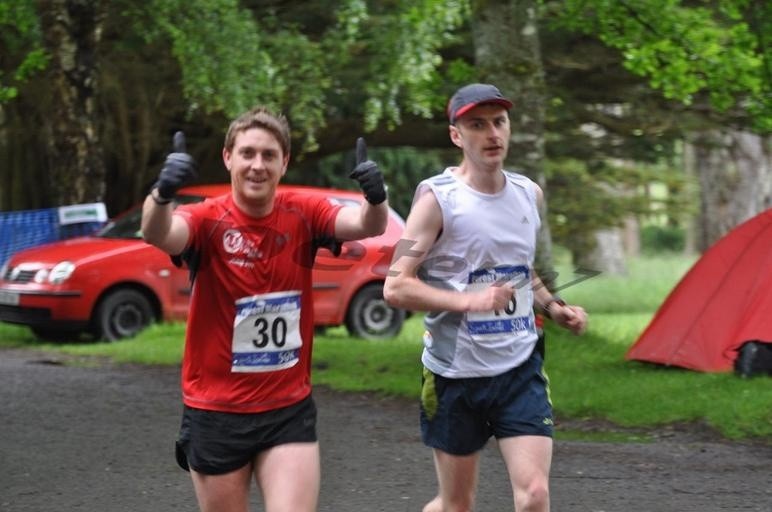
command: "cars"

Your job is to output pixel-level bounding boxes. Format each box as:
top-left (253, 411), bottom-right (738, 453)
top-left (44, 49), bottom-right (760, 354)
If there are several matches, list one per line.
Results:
top-left (1, 184), bottom-right (406, 344)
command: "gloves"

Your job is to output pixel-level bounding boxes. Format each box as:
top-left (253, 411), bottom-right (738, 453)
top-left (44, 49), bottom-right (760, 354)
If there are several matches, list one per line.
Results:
top-left (150, 129), bottom-right (198, 205)
top-left (347, 135), bottom-right (387, 207)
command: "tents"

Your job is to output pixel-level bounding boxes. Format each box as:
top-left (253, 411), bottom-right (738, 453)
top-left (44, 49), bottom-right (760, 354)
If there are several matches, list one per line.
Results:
top-left (624, 206), bottom-right (772, 374)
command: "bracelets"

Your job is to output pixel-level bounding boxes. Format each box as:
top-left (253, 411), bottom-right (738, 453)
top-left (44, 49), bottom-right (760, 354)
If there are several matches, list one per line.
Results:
top-left (542, 295), bottom-right (566, 319)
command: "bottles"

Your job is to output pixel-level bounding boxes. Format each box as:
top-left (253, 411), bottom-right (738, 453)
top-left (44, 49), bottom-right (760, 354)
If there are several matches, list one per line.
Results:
top-left (534, 313), bottom-right (545, 341)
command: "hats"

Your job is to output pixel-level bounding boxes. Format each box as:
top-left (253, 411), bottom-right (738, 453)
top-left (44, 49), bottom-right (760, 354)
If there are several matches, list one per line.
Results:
top-left (446, 81), bottom-right (513, 127)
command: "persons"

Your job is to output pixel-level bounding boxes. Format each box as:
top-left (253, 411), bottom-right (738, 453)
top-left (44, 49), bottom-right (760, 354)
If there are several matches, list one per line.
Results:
top-left (383, 82), bottom-right (590, 512)
top-left (141, 106), bottom-right (388, 512)
